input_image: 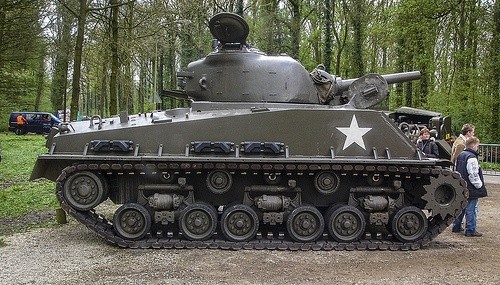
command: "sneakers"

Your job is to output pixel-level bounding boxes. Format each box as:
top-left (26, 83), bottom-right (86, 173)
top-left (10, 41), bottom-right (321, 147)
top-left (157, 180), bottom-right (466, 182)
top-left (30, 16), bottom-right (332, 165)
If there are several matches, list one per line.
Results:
top-left (465, 230), bottom-right (482, 236)
top-left (452, 227), bottom-right (464, 232)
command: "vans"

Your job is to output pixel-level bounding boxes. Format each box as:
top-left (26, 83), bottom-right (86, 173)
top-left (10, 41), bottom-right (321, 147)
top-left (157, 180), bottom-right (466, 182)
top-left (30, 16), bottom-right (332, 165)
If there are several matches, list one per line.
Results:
top-left (9, 112), bottom-right (71, 135)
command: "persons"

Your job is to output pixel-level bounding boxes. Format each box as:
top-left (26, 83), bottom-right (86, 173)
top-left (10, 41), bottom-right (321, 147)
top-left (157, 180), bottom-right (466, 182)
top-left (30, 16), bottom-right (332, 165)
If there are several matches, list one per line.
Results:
top-left (417, 128), bottom-right (439, 158)
top-left (452, 136), bottom-right (488, 237)
top-left (450, 124), bottom-right (475, 172)
top-left (17, 113), bottom-right (28, 135)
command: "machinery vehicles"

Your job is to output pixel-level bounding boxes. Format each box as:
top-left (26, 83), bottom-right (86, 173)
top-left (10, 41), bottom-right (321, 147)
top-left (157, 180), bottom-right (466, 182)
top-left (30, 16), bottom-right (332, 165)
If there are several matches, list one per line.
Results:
top-left (27, 12), bottom-right (469, 252)
